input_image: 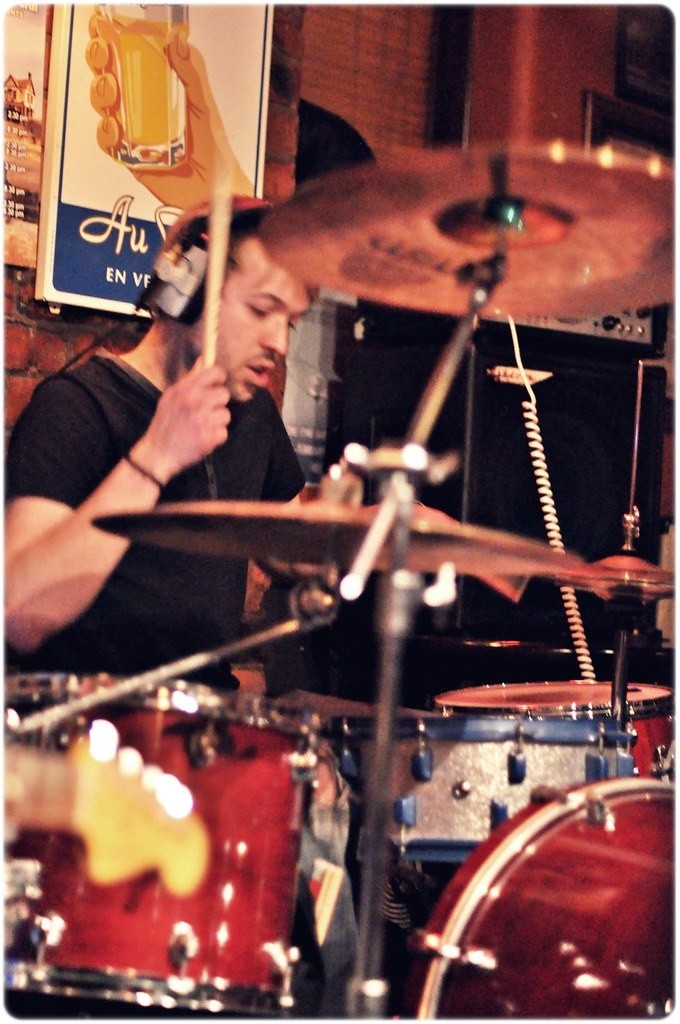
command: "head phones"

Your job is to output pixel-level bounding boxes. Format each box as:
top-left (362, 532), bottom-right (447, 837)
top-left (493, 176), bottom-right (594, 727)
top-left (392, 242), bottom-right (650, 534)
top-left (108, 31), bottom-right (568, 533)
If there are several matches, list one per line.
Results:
top-left (147, 200), bottom-right (273, 323)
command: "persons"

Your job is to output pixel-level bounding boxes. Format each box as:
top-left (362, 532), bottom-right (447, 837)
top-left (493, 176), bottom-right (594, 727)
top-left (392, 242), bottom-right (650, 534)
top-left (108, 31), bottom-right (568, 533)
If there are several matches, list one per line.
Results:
top-left (5, 196), bottom-right (421, 1020)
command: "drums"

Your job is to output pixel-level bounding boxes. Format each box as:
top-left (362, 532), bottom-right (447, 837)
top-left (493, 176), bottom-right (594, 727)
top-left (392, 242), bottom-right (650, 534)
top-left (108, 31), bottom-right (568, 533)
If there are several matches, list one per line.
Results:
top-left (350, 718), bottom-right (637, 864)
top-left (4, 671), bottom-right (313, 1019)
top-left (410, 773), bottom-right (675, 1019)
top-left (433, 680), bottom-right (673, 784)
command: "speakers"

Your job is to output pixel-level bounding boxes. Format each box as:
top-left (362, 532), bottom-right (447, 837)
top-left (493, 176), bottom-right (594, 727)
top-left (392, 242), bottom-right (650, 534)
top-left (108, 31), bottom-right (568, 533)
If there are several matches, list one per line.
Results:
top-left (303, 293), bottom-right (665, 651)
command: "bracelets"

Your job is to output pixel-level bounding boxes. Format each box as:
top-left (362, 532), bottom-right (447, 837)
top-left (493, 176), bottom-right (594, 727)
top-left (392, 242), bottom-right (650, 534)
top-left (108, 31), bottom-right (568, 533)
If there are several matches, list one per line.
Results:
top-left (123, 452), bottom-right (166, 491)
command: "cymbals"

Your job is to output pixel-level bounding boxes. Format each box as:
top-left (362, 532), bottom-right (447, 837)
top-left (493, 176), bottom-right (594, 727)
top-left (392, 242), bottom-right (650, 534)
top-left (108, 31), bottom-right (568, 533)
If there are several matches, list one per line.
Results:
top-left (553, 558), bottom-right (675, 596)
top-left (86, 499), bottom-right (595, 582)
top-left (266, 145), bottom-right (675, 324)
